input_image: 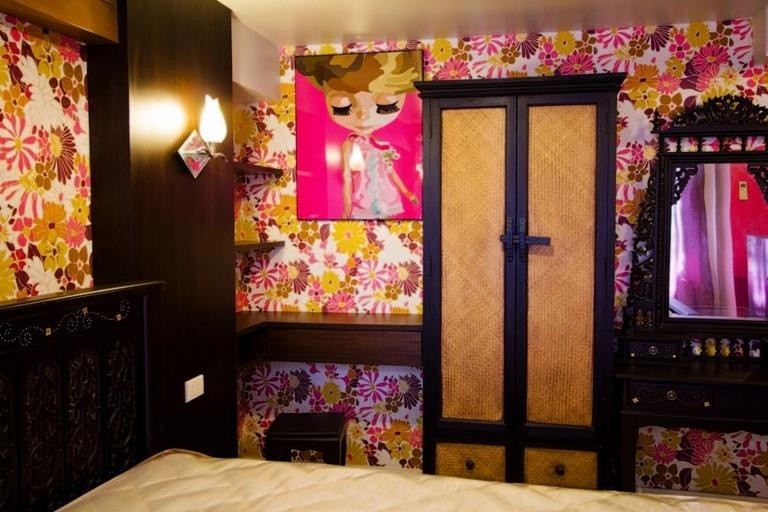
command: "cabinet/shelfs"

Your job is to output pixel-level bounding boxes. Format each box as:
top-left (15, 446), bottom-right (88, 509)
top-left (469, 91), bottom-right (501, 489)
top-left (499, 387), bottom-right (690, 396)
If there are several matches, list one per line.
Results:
top-left (232, 160), bottom-right (285, 253)
top-left (614, 353), bottom-right (768, 493)
top-left (412, 68), bottom-right (628, 490)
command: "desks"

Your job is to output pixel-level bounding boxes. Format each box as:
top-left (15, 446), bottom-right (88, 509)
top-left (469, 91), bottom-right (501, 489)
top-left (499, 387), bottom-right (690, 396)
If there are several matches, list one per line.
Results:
top-left (237, 310), bottom-right (424, 374)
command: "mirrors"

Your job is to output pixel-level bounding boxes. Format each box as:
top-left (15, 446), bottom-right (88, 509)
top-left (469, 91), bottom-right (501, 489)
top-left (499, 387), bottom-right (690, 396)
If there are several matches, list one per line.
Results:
top-left (660, 156), bottom-right (768, 331)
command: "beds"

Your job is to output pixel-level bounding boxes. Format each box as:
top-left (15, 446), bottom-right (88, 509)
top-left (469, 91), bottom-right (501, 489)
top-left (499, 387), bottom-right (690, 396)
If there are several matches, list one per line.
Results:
top-left (52, 447), bottom-right (767, 510)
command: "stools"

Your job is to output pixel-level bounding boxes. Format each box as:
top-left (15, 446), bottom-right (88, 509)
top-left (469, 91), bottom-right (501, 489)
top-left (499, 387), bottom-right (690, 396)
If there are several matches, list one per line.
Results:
top-left (262, 413), bottom-right (347, 466)
